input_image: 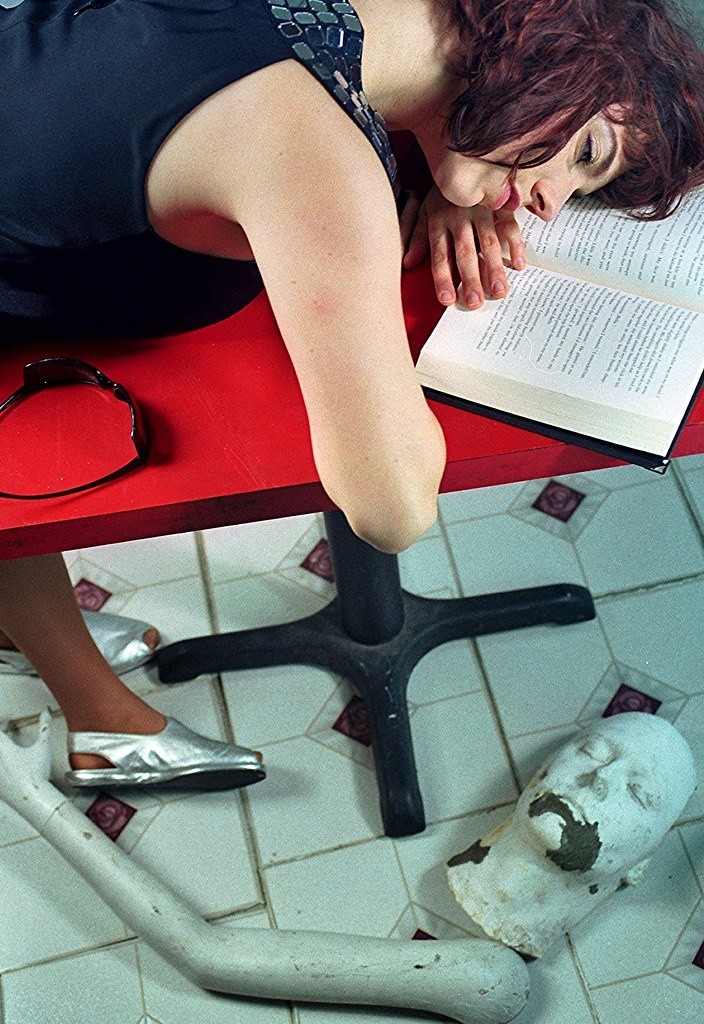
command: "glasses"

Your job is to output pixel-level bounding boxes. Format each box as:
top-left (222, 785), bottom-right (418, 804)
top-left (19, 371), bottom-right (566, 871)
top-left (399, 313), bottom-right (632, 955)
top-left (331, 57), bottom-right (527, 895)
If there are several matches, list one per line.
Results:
top-left (0, 358), bottom-right (149, 500)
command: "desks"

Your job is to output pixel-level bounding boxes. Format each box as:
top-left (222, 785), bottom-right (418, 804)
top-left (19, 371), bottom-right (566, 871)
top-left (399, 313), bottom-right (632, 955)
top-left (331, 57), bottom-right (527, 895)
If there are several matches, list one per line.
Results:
top-left (0, 246), bottom-right (704, 839)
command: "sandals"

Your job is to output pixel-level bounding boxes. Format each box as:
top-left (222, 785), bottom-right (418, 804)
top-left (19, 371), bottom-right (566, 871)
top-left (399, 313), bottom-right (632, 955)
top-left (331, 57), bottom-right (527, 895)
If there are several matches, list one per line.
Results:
top-left (0, 610), bottom-right (164, 676)
top-left (65, 717), bottom-right (266, 791)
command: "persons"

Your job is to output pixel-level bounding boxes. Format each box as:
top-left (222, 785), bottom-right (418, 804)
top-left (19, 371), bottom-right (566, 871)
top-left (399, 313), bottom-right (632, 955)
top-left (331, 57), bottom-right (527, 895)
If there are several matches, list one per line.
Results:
top-left (445, 712), bottom-right (698, 957)
top-left (0, 0), bottom-right (704, 798)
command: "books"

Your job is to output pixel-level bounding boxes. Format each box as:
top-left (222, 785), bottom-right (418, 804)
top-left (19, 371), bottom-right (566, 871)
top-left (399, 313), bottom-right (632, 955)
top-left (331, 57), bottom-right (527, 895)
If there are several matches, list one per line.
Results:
top-left (414, 182), bottom-right (704, 475)
